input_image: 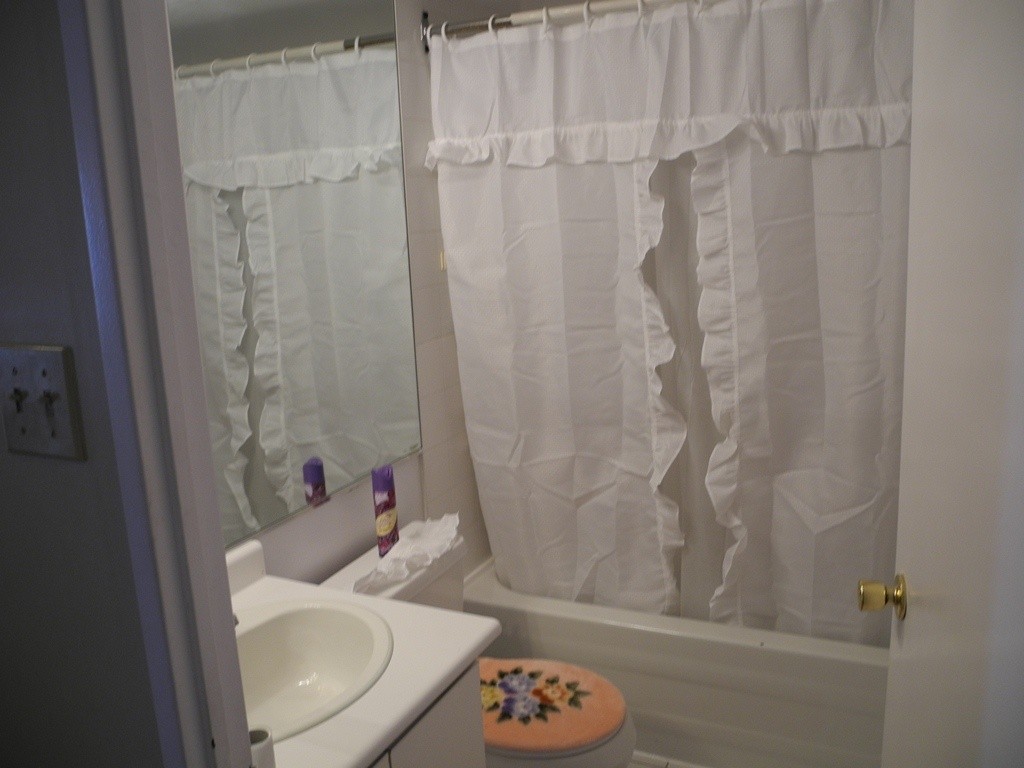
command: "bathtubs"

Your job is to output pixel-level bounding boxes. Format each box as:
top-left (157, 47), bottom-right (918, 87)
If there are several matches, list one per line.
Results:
top-left (462, 552), bottom-right (891, 768)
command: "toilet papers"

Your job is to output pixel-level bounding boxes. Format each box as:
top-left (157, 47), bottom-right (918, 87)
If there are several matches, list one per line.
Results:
top-left (247, 727), bottom-right (277, 768)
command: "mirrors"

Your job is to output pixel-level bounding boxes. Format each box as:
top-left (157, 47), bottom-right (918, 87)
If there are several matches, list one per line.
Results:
top-left (165, 0), bottom-right (423, 556)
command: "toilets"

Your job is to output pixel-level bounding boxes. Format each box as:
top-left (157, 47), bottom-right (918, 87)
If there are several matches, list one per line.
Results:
top-left (316, 518), bottom-right (641, 768)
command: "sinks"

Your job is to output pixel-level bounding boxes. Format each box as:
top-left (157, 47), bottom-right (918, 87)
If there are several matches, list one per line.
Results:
top-left (235, 599), bottom-right (393, 743)
